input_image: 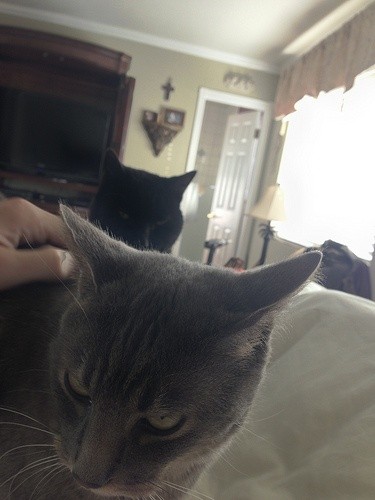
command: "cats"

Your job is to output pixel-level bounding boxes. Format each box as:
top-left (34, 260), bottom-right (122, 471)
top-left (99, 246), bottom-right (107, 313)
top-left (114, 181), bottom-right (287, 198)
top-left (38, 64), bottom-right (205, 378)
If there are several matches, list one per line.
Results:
top-left (0, 198), bottom-right (325, 500)
top-left (290, 237), bottom-right (371, 299)
top-left (92, 149), bottom-right (200, 264)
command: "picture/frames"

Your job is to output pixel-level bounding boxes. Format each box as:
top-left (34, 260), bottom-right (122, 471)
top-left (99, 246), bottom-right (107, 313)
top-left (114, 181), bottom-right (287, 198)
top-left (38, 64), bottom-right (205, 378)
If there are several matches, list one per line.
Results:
top-left (157, 106), bottom-right (186, 132)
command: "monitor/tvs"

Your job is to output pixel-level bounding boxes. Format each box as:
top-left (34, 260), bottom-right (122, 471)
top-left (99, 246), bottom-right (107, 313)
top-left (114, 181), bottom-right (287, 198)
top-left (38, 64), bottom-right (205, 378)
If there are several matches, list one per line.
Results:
top-left (0, 41), bottom-right (136, 202)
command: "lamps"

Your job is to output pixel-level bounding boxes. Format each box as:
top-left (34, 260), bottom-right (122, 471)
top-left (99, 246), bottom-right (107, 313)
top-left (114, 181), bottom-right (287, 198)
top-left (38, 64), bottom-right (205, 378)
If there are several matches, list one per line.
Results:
top-left (247, 183), bottom-right (287, 267)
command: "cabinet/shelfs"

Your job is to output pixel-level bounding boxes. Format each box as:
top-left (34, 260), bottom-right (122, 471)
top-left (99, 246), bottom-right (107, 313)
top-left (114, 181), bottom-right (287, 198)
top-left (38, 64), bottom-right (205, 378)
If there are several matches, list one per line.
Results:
top-left (0, 25), bottom-right (136, 219)
